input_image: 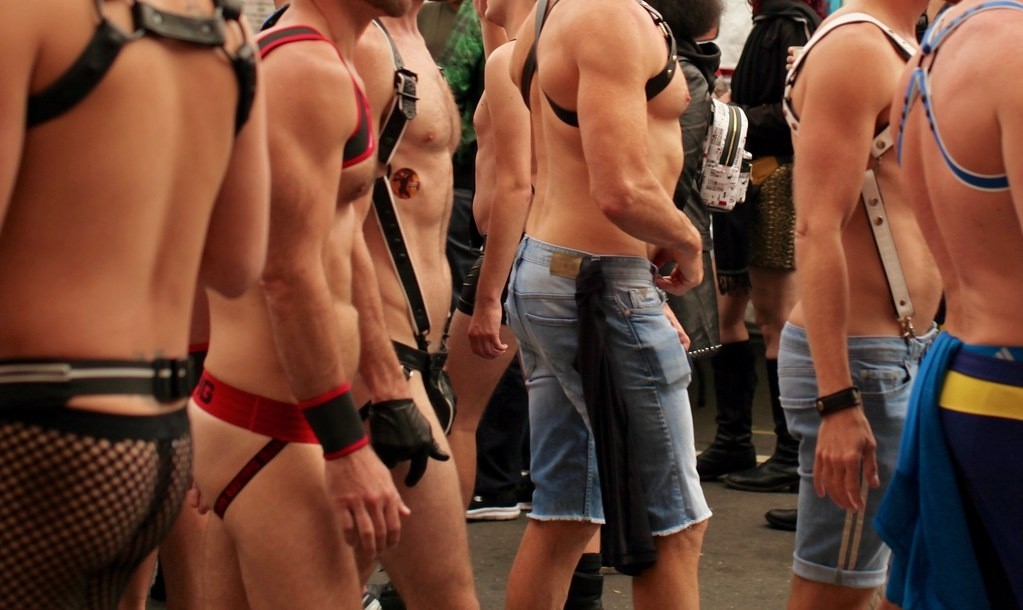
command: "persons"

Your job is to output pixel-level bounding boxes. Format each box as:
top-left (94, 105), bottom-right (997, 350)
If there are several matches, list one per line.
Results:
top-left (1, 0), bottom-right (1023, 609)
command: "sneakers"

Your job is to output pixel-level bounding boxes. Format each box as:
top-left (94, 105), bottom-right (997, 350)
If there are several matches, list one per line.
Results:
top-left (465, 471), bottom-right (535, 521)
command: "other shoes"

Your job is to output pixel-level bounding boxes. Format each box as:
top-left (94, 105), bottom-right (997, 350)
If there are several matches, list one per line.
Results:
top-left (362, 580), bottom-right (406, 610)
top-left (565, 571), bottom-right (603, 610)
top-left (766, 509), bottom-right (798, 531)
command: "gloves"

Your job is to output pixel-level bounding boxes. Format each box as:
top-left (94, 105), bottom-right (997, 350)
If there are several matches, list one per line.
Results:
top-left (369, 399), bottom-right (449, 487)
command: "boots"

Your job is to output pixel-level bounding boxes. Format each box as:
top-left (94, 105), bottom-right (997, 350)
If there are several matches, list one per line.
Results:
top-left (696, 338), bottom-right (757, 480)
top-left (725, 358), bottom-right (800, 493)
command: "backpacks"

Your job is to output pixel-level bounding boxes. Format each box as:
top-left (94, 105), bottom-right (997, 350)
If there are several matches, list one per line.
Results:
top-left (678, 53), bottom-right (753, 214)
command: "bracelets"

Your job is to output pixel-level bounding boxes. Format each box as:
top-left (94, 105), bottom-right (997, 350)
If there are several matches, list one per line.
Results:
top-left (813, 386), bottom-right (861, 418)
top-left (298, 381), bottom-right (369, 460)
